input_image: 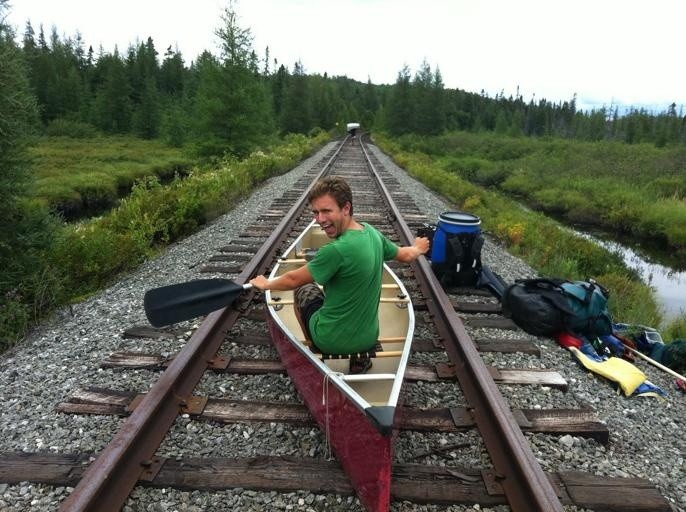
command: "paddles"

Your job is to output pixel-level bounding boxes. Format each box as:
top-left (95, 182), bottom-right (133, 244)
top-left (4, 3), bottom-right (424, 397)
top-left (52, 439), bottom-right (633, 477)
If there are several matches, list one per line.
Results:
top-left (140, 276), bottom-right (282, 329)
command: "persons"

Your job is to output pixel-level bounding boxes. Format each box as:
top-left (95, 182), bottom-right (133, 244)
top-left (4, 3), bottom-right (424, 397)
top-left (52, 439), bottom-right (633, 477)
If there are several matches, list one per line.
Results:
top-left (348, 129), bottom-right (357, 146)
top-left (249, 176), bottom-right (430, 375)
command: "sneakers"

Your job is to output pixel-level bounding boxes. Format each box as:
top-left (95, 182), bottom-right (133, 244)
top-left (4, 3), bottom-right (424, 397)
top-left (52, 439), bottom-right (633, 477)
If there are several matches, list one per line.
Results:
top-left (348, 357), bottom-right (372, 375)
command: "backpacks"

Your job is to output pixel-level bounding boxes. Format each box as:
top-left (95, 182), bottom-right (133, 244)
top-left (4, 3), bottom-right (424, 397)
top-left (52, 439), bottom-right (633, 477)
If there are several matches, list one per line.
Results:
top-left (501, 277), bottom-right (608, 338)
